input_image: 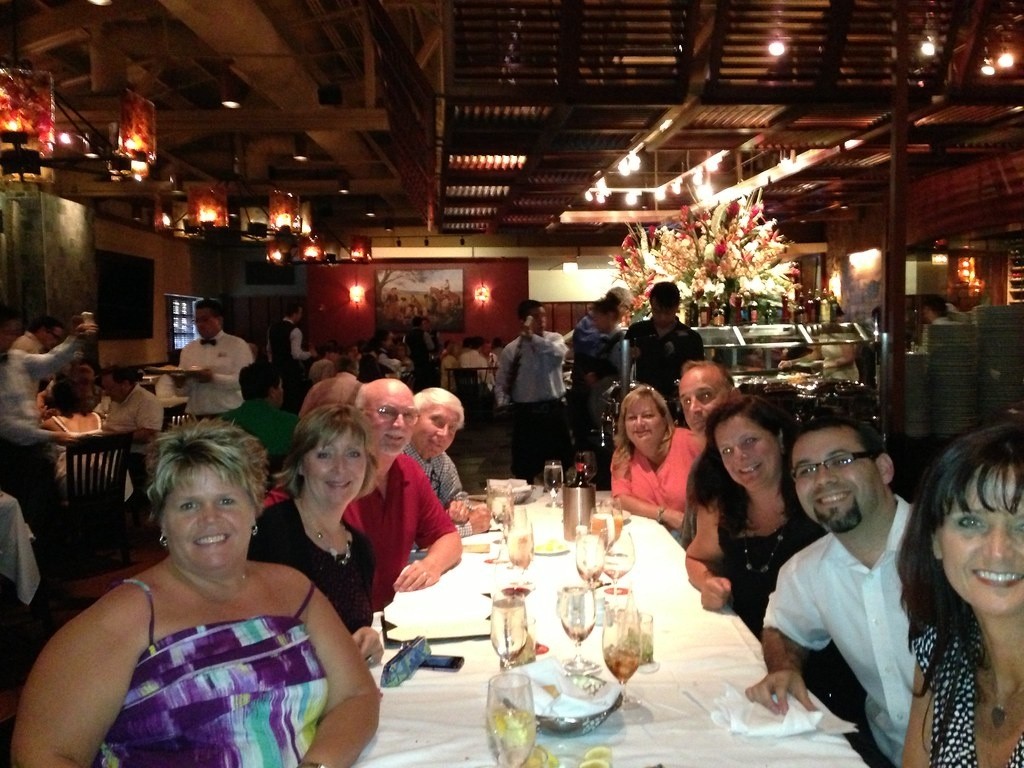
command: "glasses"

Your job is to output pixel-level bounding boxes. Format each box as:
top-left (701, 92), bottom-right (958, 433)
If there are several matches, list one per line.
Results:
top-left (49, 329), bottom-right (63, 342)
top-left (791, 450), bottom-right (874, 483)
top-left (361, 405), bottom-right (420, 426)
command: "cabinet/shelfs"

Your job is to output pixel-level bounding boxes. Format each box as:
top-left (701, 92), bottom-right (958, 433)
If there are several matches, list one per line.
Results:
top-left (1006, 245), bottom-right (1024, 305)
top-left (688, 323), bottom-right (875, 391)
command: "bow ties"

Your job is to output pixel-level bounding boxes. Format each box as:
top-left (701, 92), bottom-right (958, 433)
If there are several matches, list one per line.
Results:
top-left (200, 339), bottom-right (217, 346)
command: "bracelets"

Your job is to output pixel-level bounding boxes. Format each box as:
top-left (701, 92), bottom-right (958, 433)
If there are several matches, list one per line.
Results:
top-left (658, 506), bottom-right (665, 522)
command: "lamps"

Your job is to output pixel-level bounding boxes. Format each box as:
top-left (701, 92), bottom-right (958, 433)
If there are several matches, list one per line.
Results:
top-left (0, 68), bottom-right (465, 266)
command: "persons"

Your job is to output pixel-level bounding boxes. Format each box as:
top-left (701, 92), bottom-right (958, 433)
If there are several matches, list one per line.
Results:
top-left (490, 279), bottom-right (957, 492)
top-left (683, 395), bottom-right (867, 730)
top-left (259, 378), bottom-right (462, 615)
top-left (897, 423), bottom-right (1024, 768)
top-left (245, 404), bottom-right (383, 670)
top-left (747, 417), bottom-right (916, 768)
top-left (8, 419), bottom-right (382, 768)
top-left (268, 302), bottom-right (504, 416)
top-left (401, 387), bottom-right (471, 555)
top-left (665, 354), bottom-right (734, 542)
top-left (607, 383), bottom-right (707, 544)
top-left (0, 298), bottom-right (295, 532)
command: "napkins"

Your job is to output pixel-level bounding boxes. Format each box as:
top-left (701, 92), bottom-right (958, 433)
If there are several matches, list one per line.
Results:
top-left (715, 676), bottom-right (859, 738)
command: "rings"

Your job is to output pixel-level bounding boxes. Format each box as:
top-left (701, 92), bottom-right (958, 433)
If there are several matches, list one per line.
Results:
top-left (366, 657), bottom-right (374, 664)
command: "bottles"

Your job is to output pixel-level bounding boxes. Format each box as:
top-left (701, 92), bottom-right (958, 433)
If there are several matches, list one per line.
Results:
top-left (688, 285), bottom-right (841, 327)
top-left (563, 459), bottom-right (596, 542)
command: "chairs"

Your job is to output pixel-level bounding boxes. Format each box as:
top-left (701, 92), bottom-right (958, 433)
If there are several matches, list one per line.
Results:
top-left (45, 431), bottom-right (138, 578)
top-left (401, 358), bottom-right (499, 429)
top-left (162, 402), bottom-right (194, 432)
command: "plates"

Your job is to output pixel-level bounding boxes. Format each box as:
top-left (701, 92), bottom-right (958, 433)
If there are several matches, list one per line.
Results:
top-left (532, 545), bottom-right (569, 555)
top-left (905, 304), bottom-right (1024, 437)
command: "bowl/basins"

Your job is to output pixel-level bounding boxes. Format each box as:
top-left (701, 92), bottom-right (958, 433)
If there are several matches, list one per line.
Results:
top-left (487, 485), bottom-right (535, 505)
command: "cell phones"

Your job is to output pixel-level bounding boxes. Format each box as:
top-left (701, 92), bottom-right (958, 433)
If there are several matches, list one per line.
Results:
top-left (419, 654), bottom-right (465, 669)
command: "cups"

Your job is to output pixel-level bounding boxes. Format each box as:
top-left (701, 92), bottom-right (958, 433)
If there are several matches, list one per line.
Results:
top-left (544, 461), bottom-right (564, 510)
top-left (638, 614), bottom-right (654, 664)
top-left (497, 746), bottom-right (549, 768)
top-left (499, 615), bottom-right (536, 670)
top-left (576, 452), bottom-right (597, 482)
top-left (486, 674), bottom-right (537, 768)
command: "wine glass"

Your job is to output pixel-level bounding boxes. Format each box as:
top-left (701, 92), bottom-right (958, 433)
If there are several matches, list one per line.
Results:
top-left (557, 586), bottom-right (603, 677)
top-left (573, 498), bottom-right (636, 624)
top-left (601, 606), bottom-right (643, 711)
top-left (486, 484), bottom-right (534, 591)
top-left (491, 594), bottom-right (527, 673)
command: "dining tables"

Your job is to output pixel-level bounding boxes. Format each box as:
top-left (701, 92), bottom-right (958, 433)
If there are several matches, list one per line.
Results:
top-left (341, 487), bottom-right (875, 768)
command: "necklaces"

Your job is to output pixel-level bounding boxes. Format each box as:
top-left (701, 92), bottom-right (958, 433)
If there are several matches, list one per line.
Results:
top-left (301, 510), bottom-right (339, 549)
top-left (734, 533), bottom-right (786, 574)
top-left (989, 706), bottom-right (1006, 729)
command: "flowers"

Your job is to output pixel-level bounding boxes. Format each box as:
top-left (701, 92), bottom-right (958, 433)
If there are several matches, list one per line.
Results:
top-left (604, 185), bottom-right (804, 313)
